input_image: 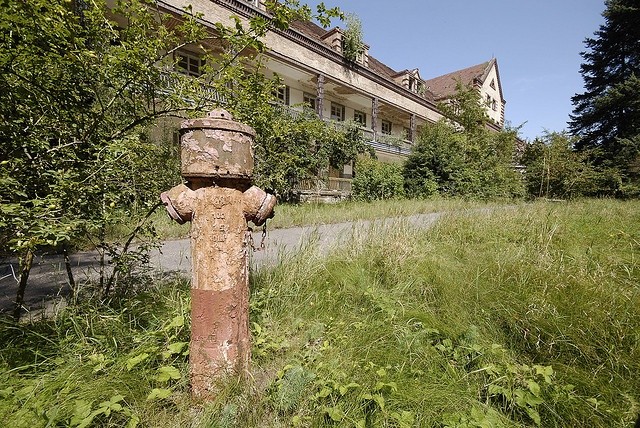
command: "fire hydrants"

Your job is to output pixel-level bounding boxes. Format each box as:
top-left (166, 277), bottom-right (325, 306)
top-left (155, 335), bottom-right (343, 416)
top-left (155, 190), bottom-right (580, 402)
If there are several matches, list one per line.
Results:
top-left (159, 106), bottom-right (278, 402)
